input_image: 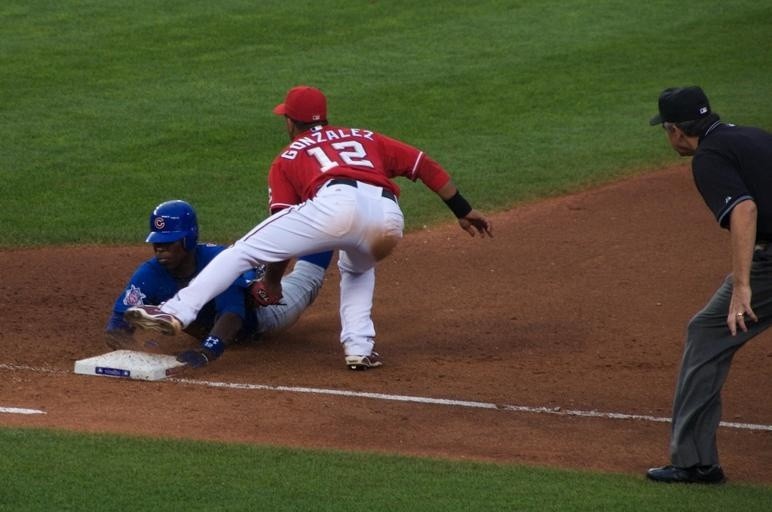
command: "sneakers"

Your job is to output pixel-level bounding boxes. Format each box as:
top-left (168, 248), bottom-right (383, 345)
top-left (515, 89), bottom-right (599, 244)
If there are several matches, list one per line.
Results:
top-left (124, 305), bottom-right (182, 337)
top-left (647, 462), bottom-right (723, 483)
top-left (345, 351), bottom-right (382, 369)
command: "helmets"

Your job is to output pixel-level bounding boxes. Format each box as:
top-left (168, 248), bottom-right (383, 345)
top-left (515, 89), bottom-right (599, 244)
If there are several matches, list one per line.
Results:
top-left (145, 199), bottom-right (199, 252)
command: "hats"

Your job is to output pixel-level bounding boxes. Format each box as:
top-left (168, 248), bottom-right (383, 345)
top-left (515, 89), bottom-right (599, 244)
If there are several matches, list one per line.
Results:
top-left (273, 87), bottom-right (327, 123)
top-left (648, 85), bottom-right (711, 126)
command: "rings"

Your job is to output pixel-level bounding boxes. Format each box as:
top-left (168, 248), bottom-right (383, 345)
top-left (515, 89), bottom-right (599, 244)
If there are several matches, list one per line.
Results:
top-left (736, 312), bottom-right (744, 317)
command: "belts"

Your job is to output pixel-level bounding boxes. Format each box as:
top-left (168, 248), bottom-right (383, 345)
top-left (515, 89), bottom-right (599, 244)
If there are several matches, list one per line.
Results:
top-left (327, 177), bottom-right (396, 201)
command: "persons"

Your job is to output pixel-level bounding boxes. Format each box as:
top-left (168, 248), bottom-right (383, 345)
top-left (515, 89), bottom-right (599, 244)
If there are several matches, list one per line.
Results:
top-left (124, 84), bottom-right (495, 369)
top-left (105, 199), bottom-right (334, 370)
top-left (645, 84), bottom-right (772, 486)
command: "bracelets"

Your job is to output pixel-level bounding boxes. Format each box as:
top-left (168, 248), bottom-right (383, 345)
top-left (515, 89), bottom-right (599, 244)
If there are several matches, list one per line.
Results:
top-left (443, 189), bottom-right (473, 220)
top-left (200, 335), bottom-right (224, 359)
